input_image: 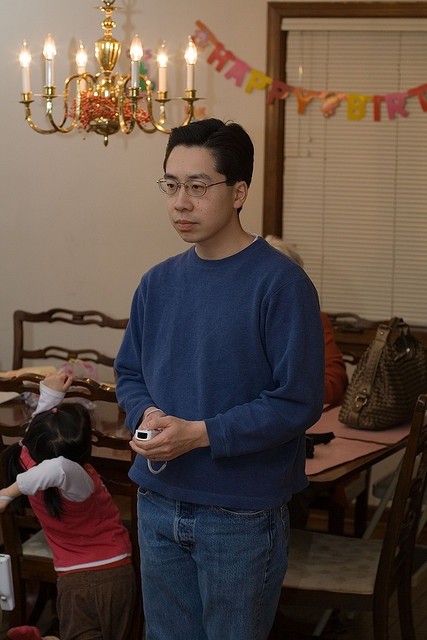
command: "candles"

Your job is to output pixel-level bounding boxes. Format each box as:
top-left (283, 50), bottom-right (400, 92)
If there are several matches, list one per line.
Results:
top-left (41, 31), bottom-right (57, 86)
top-left (183, 34), bottom-right (199, 88)
top-left (19, 38), bottom-right (31, 95)
top-left (128, 30), bottom-right (143, 87)
top-left (74, 43), bottom-right (87, 110)
top-left (156, 37), bottom-right (169, 91)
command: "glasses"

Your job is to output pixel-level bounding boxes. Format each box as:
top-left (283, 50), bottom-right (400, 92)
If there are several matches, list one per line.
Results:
top-left (156, 177), bottom-right (227, 197)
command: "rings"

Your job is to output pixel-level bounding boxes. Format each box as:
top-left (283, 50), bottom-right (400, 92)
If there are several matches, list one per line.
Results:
top-left (152, 454), bottom-right (155, 459)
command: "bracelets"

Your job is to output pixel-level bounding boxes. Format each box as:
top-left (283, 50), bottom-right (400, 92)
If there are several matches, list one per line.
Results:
top-left (144, 408), bottom-right (166, 418)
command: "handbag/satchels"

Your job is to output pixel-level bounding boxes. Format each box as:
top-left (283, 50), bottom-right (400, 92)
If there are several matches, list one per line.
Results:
top-left (338, 317), bottom-right (427, 430)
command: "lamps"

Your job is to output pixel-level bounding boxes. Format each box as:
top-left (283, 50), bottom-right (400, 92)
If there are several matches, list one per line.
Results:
top-left (16, 0), bottom-right (205, 148)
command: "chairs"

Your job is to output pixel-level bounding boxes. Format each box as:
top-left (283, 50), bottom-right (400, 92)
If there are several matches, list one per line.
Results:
top-left (11, 307), bottom-right (130, 371)
top-left (326, 310), bottom-right (378, 366)
top-left (270, 395), bottom-right (427, 640)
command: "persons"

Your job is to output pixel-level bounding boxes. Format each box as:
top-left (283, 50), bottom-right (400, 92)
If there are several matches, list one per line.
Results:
top-left (111, 117), bottom-right (326, 640)
top-left (263, 233), bottom-right (349, 404)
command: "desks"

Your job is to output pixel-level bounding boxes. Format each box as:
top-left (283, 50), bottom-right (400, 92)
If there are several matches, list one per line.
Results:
top-left (1, 399), bottom-right (409, 536)
